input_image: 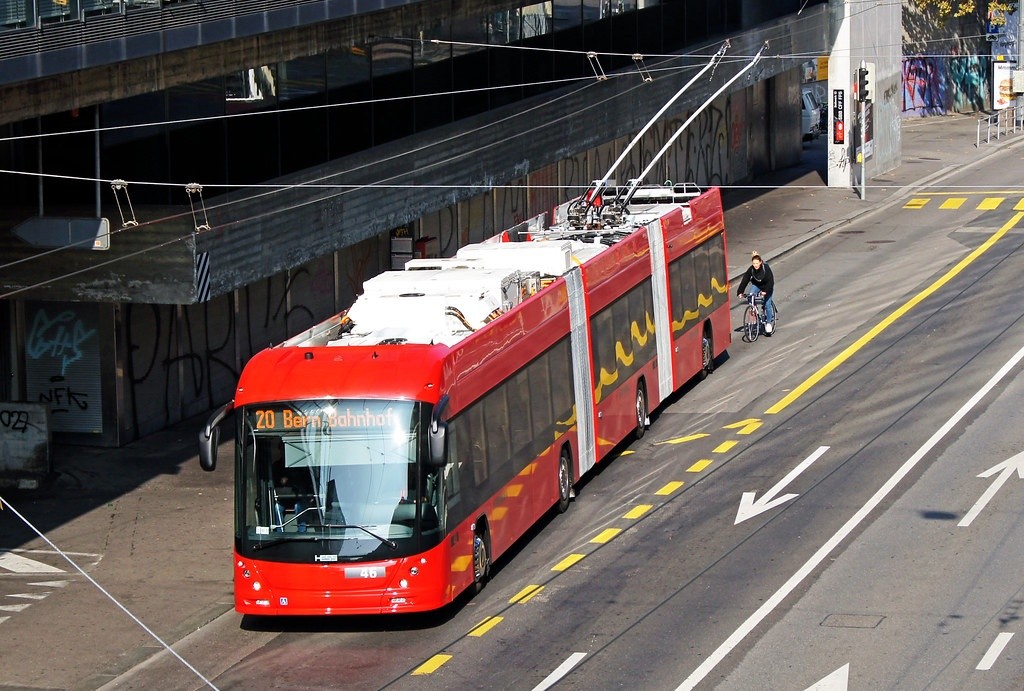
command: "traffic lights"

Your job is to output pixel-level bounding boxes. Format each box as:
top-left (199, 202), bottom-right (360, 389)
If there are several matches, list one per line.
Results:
top-left (857, 67), bottom-right (868, 102)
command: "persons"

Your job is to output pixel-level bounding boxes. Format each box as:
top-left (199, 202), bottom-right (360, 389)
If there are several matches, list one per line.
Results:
top-left (272, 442), bottom-right (316, 532)
top-left (737, 250), bottom-right (774, 333)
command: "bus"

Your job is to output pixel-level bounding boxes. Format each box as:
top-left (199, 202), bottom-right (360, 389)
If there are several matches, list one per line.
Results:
top-left (199, 40), bottom-right (770, 618)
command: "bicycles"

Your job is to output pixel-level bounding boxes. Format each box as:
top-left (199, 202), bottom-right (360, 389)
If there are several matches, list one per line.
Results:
top-left (737, 294), bottom-right (778, 342)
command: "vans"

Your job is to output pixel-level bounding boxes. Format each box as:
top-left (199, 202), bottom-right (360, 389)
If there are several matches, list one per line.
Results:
top-left (801, 90), bottom-right (822, 141)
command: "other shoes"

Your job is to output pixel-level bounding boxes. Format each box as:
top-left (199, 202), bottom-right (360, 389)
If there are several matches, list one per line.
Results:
top-left (765, 323), bottom-right (772, 332)
